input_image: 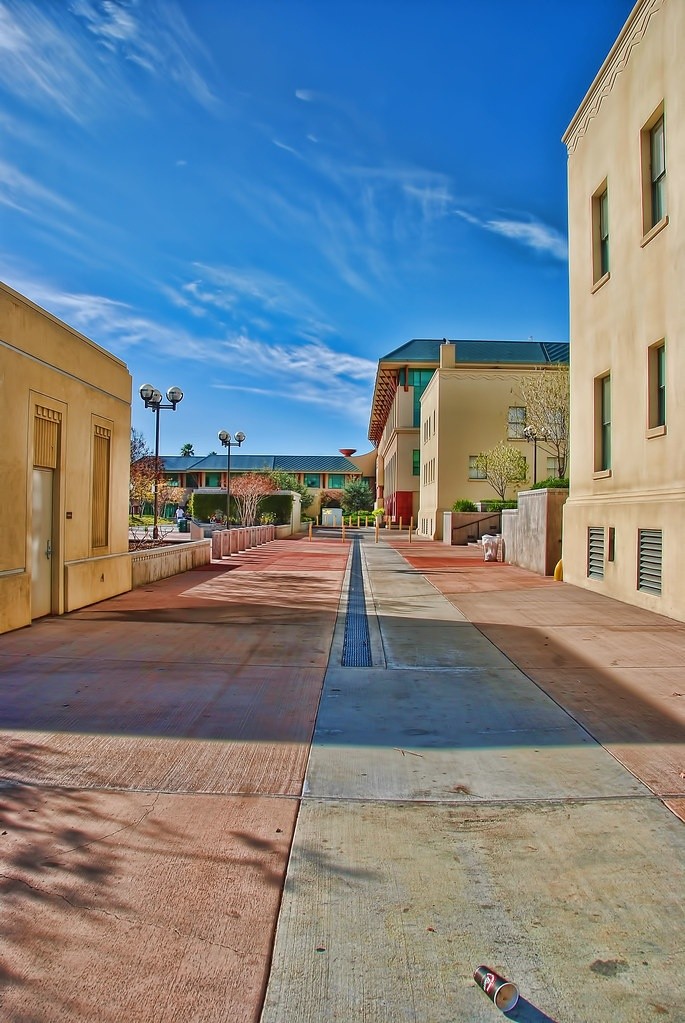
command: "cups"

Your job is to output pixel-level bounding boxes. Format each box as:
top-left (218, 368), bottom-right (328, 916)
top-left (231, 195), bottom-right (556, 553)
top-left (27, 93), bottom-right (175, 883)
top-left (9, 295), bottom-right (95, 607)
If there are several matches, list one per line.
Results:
top-left (474, 965), bottom-right (520, 1012)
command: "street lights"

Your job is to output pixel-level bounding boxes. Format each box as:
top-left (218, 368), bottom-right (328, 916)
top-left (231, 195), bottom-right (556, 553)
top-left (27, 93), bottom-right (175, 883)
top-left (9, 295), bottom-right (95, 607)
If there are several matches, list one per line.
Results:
top-left (218, 429), bottom-right (246, 528)
top-left (139, 383), bottom-right (183, 539)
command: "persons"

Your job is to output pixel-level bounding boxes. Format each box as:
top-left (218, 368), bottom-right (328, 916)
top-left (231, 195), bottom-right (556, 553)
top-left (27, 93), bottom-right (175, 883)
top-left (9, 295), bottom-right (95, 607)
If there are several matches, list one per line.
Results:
top-left (176, 506), bottom-right (184, 519)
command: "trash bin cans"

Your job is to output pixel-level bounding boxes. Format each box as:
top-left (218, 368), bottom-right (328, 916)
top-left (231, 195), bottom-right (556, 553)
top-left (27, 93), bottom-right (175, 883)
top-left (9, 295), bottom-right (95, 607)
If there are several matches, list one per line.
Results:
top-left (178, 518), bottom-right (187, 533)
top-left (482, 534), bottom-right (500, 562)
